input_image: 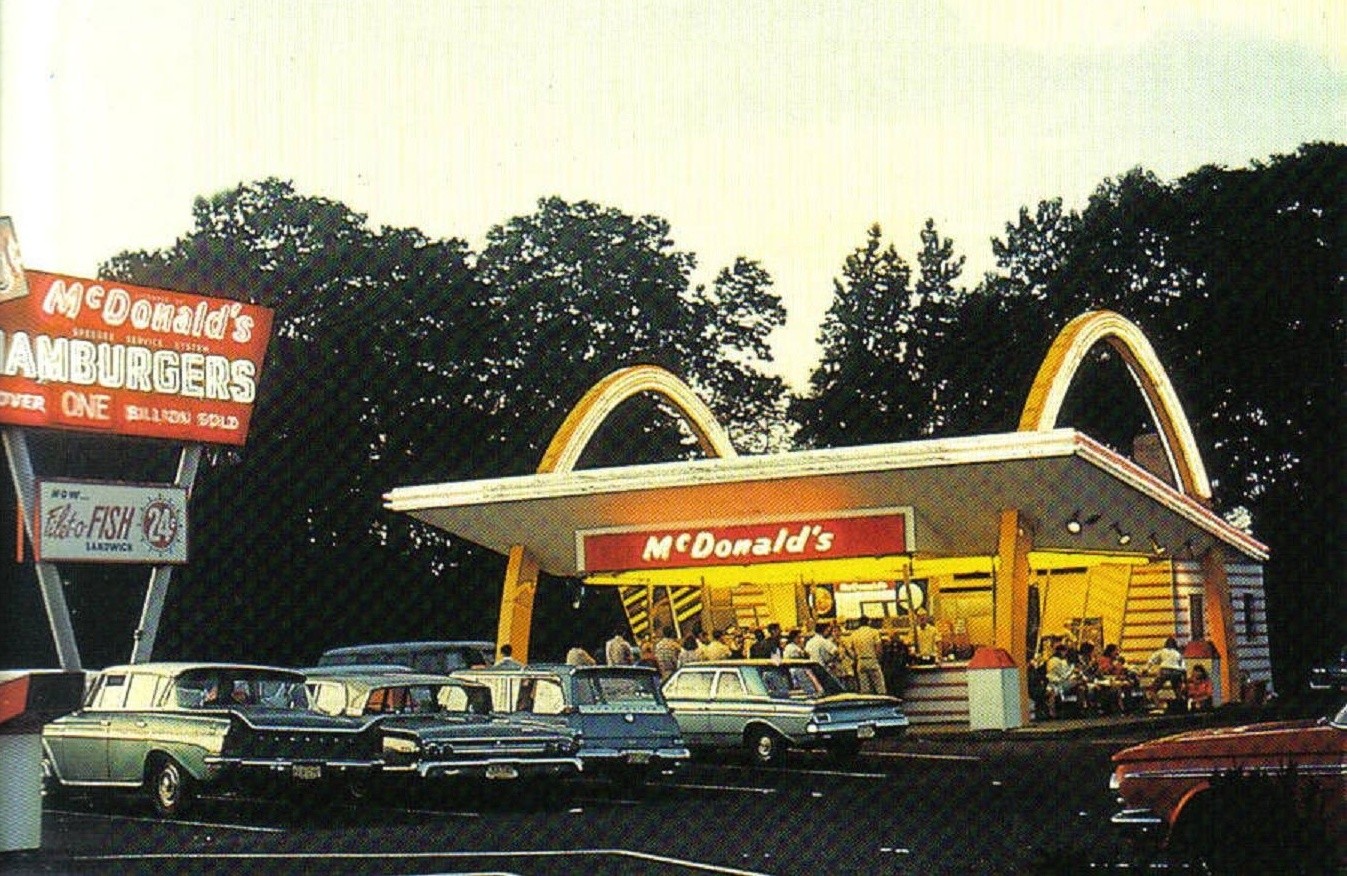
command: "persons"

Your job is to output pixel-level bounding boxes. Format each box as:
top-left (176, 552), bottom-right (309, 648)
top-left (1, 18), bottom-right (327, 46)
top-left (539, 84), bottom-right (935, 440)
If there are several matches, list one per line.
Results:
top-left (1042, 634), bottom-right (1130, 718)
top-left (907, 607), bottom-right (942, 660)
top-left (491, 645), bottom-right (523, 670)
top-left (1139, 636), bottom-right (1214, 716)
top-left (566, 639), bottom-right (596, 667)
top-left (605, 616), bottom-right (913, 698)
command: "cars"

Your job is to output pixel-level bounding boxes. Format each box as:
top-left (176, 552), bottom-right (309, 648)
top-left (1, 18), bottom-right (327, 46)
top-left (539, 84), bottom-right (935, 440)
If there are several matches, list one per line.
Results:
top-left (658, 658), bottom-right (911, 771)
top-left (36, 660), bottom-right (388, 821)
top-left (1107, 702), bottom-right (1347, 876)
top-left (298, 638), bottom-right (585, 814)
top-left (433, 656), bottom-right (692, 801)
top-left (1307, 641), bottom-right (1347, 693)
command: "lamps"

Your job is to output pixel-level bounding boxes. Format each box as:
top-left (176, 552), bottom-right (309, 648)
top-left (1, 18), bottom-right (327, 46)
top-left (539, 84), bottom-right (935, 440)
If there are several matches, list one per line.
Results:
top-left (1066, 511), bottom-right (1167, 555)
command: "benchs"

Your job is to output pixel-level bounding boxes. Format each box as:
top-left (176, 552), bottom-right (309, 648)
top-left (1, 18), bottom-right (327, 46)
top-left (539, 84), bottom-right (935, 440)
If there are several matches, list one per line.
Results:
top-left (1060, 686), bottom-right (1143, 701)
